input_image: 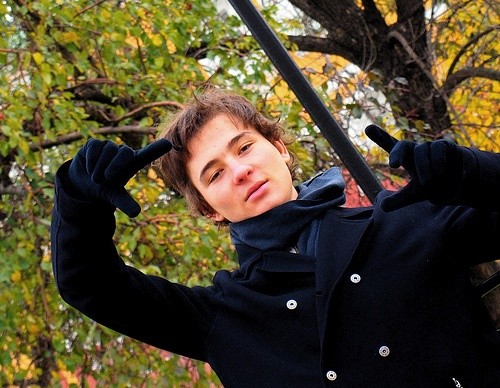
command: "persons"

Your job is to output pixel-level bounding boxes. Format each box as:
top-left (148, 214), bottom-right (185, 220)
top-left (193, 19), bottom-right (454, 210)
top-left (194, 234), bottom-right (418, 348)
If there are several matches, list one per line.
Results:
top-left (51, 95), bottom-right (500, 388)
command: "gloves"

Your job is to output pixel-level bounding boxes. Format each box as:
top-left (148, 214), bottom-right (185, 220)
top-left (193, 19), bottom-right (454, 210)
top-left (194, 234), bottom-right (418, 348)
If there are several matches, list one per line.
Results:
top-left (365, 123), bottom-right (477, 214)
top-left (66, 137), bottom-right (173, 218)
